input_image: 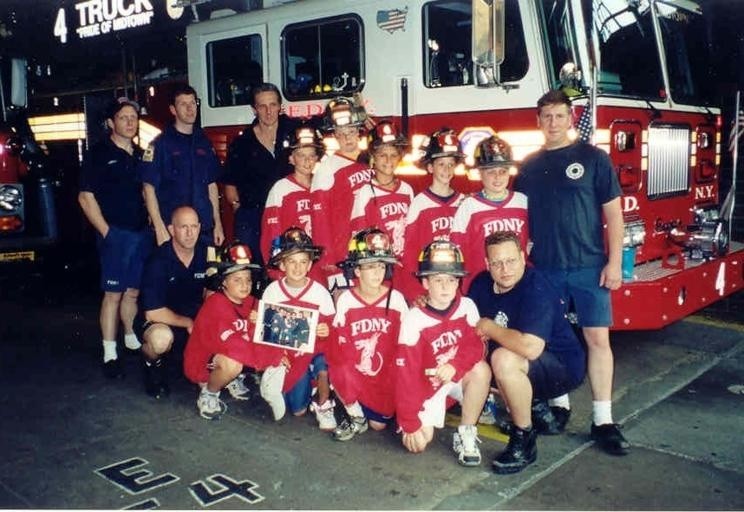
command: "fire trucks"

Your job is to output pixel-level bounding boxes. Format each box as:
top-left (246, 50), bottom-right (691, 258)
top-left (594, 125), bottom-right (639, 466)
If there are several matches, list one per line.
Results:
top-left (0, 0), bottom-right (80, 281)
top-left (185, 2), bottom-right (744, 335)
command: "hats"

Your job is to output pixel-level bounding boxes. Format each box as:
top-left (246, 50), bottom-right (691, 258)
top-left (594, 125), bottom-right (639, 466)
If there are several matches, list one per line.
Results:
top-left (108, 96), bottom-right (140, 114)
top-left (267, 224), bottom-right (322, 265)
top-left (259, 364), bottom-right (288, 422)
top-left (413, 239), bottom-right (471, 277)
top-left (418, 126), bottom-right (468, 162)
top-left (366, 118), bottom-right (412, 150)
top-left (213, 238), bottom-right (265, 276)
top-left (324, 95), bottom-right (363, 131)
top-left (472, 135), bottom-right (519, 168)
top-left (347, 227), bottom-right (404, 269)
top-left (285, 125), bottom-right (324, 153)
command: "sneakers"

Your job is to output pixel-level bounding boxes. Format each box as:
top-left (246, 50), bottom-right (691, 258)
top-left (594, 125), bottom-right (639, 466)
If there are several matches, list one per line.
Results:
top-left (491, 424), bottom-right (538, 475)
top-left (196, 382), bottom-right (223, 421)
top-left (141, 366), bottom-right (171, 401)
top-left (224, 377), bottom-right (252, 403)
top-left (540, 405), bottom-right (572, 436)
top-left (102, 356), bottom-right (127, 383)
top-left (451, 424), bottom-right (482, 466)
top-left (590, 419), bottom-right (631, 457)
top-left (311, 398), bottom-right (339, 434)
top-left (331, 414), bottom-right (370, 443)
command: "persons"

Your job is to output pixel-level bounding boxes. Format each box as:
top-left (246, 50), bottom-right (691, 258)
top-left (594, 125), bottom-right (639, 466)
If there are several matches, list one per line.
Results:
top-left (224, 84), bottom-right (299, 387)
top-left (252, 226), bottom-right (337, 431)
top-left (77, 96), bottom-right (153, 379)
top-left (262, 98), bottom-right (530, 301)
top-left (324, 228), bottom-right (410, 440)
top-left (136, 87), bottom-right (226, 250)
top-left (132, 205), bottom-right (223, 399)
top-left (183, 239), bottom-right (292, 421)
top-left (512, 92), bottom-right (631, 456)
top-left (394, 241), bottom-right (492, 463)
top-left (407, 231), bottom-right (587, 473)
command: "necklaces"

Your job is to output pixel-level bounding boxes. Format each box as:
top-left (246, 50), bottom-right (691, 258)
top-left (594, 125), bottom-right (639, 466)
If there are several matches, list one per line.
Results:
top-left (481, 188), bottom-right (509, 200)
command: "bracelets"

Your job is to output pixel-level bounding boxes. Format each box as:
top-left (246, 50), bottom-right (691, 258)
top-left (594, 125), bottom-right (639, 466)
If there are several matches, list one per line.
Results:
top-left (230, 200), bottom-right (239, 205)
